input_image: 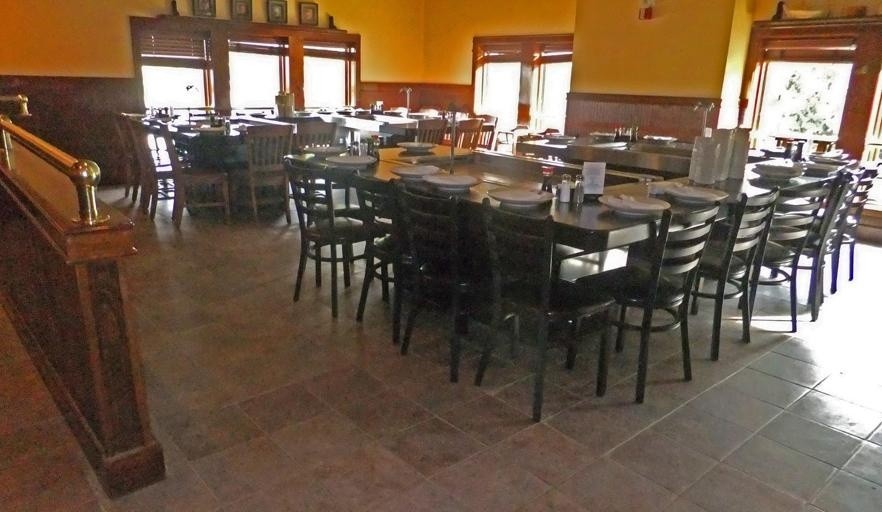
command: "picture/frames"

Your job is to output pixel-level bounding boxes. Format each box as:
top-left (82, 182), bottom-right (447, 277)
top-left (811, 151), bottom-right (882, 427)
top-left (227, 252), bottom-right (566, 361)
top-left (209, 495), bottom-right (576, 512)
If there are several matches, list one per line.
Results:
top-left (192, 0), bottom-right (217, 18)
top-left (300, 2), bottom-right (319, 26)
top-left (267, 0), bottom-right (288, 24)
top-left (230, 1), bottom-right (253, 21)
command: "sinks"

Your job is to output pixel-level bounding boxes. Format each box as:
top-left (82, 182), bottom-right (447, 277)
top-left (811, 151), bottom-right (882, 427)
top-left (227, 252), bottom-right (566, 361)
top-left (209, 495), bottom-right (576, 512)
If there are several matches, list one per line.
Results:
top-left (186, 117), bottom-right (275, 135)
top-left (342, 114), bottom-right (417, 132)
top-left (373, 111), bottom-right (448, 130)
top-left (589, 141), bottom-right (773, 177)
top-left (380, 151), bottom-right (665, 197)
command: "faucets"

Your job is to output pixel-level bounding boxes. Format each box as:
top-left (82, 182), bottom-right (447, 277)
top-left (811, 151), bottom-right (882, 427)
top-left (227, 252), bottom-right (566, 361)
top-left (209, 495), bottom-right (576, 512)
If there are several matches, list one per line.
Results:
top-left (186, 83), bottom-right (199, 123)
top-left (693, 101), bottom-right (715, 135)
top-left (437, 101), bottom-right (476, 175)
top-left (399, 86), bottom-right (413, 113)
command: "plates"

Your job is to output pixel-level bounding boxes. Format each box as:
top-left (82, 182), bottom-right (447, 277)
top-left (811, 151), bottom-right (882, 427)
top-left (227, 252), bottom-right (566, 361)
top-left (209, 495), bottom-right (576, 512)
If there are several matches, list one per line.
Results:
top-left (487, 187), bottom-right (555, 208)
top-left (794, 162), bottom-right (839, 177)
top-left (302, 147), bottom-right (483, 196)
top-left (541, 131), bottom-right (680, 148)
top-left (395, 140), bottom-right (437, 153)
top-left (752, 166), bottom-right (802, 181)
top-left (665, 184), bottom-right (730, 204)
top-left (598, 193), bottom-right (672, 217)
top-left (197, 127), bottom-right (224, 135)
top-left (251, 112), bottom-right (267, 118)
top-left (762, 147), bottom-right (785, 157)
top-left (295, 108), bottom-right (427, 118)
top-left (689, 125), bottom-right (753, 187)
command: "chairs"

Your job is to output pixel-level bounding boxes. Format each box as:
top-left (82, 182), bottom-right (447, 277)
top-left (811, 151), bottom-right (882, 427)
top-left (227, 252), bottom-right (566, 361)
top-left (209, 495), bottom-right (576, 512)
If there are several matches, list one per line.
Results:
top-left (567, 198), bottom-right (722, 403)
top-left (392, 179), bottom-right (532, 382)
top-left (110, 107), bottom-right (498, 225)
top-left (284, 125), bottom-right (878, 344)
top-left (676, 185), bottom-right (780, 361)
top-left (475, 196), bottom-right (612, 420)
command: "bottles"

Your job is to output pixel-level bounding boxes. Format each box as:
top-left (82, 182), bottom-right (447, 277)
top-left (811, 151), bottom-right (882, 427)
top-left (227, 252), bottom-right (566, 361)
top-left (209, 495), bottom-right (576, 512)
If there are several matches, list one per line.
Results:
top-left (614, 127), bottom-right (639, 142)
top-left (539, 164), bottom-right (553, 194)
top-left (784, 141), bottom-right (793, 159)
top-left (573, 175), bottom-right (586, 206)
top-left (560, 174), bottom-right (571, 202)
top-left (213, 111), bottom-right (224, 127)
top-left (352, 134), bottom-right (379, 166)
top-left (225, 118), bottom-right (230, 134)
top-left (794, 141), bottom-right (805, 163)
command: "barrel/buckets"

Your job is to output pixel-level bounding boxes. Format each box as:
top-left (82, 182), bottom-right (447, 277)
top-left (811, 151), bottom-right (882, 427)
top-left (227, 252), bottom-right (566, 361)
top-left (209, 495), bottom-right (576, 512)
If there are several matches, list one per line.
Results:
top-left (274, 92), bottom-right (295, 117)
